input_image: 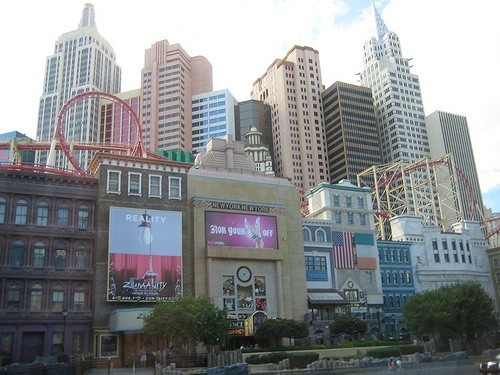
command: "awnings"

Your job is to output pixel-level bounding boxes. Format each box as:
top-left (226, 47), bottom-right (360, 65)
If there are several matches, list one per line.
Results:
top-left (307, 289), bottom-right (349, 305)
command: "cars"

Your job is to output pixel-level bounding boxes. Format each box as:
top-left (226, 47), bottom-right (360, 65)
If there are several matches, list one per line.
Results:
top-left (478, 353), bottom-right (500, 375)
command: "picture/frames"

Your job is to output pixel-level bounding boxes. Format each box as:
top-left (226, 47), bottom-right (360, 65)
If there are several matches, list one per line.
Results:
top-left (254, 275), bottom-right (267, 296)
top-left (223, 297), bottom-right (235, 311)
top-left (255, 297), bottom-right (268, 312)
top-left (222, 274), bottom-right (235, 296)
top-left (106, 205), bottom-right (184, 303)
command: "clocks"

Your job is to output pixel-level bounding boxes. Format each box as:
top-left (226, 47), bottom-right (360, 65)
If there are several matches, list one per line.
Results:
top-left (237, 266), bottom-right (252, 283)
top-left (347, 281), bottom-right (354, 288)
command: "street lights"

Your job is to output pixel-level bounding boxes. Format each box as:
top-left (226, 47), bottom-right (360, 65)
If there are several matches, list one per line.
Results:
top-left (391, 313), bottom-right (397, 333)
top-left (61, 307), bottom-right (70, 353)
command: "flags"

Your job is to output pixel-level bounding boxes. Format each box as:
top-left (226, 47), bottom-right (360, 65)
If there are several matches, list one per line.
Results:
top-left (354, 233), bottom-right (377, 269)
top-left (332, 231), bottom-right (355, 269)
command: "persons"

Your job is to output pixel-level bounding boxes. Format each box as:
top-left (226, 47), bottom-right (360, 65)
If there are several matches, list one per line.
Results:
top-left (240, 343), bottom-right (260, 350)
top-left (388, 359), bottom-right (394, 367)
top-left (245, 216), bottom-right (264, 248)
top-left (140, 345), bottom-right (147, 368)
top-left (394, 357), bottom-right (402, 367)
